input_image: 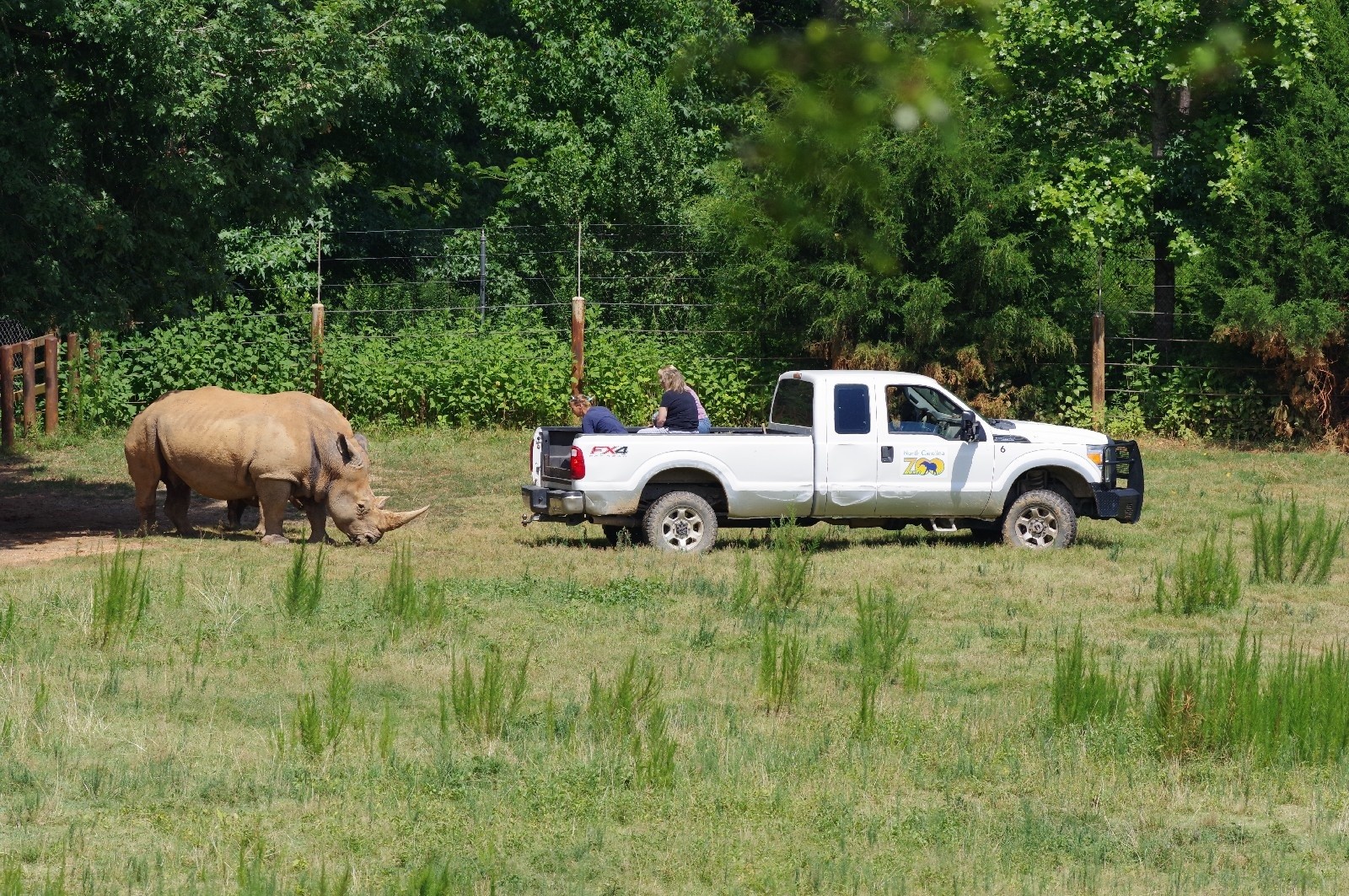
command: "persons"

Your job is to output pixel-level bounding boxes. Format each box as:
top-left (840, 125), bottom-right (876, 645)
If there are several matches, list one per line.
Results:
top-left (568, 394), bottom-right (628, 435)
top-left (682, 375), bottom-right (711, 434)
top-left (636, 365), bottom-right (699, 435)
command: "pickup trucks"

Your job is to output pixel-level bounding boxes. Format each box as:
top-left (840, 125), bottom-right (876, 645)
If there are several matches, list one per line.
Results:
top-left (520, 369), bottom-right (1146, 559)
